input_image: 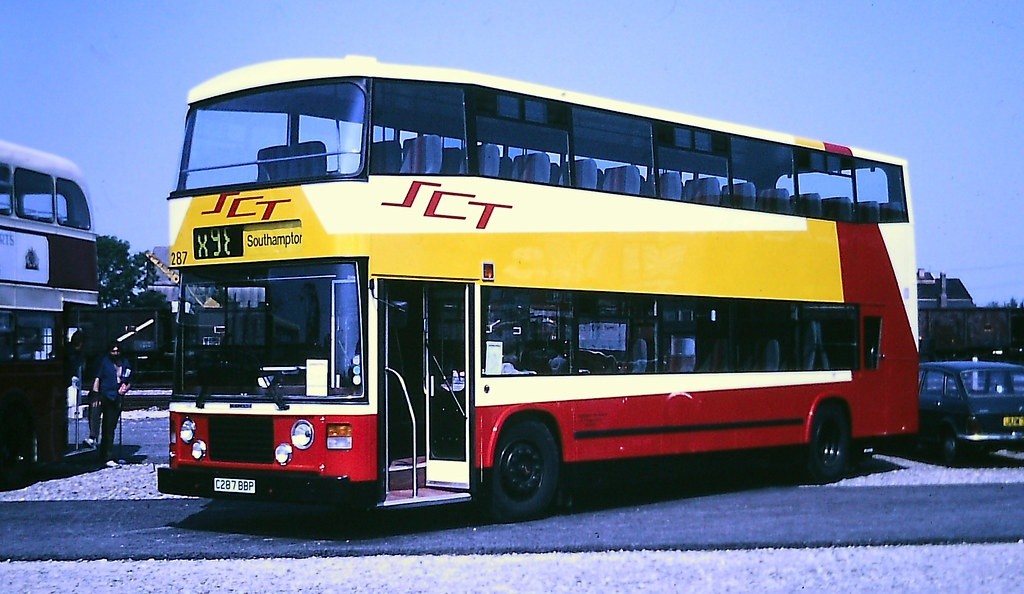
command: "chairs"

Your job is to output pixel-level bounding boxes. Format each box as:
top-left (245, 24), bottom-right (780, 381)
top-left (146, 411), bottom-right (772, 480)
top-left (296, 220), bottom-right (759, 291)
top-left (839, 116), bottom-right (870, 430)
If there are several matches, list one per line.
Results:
top-left (254, 135), bottom-right (906, 226)
top-left (633, 323), bottom-right (830, 372)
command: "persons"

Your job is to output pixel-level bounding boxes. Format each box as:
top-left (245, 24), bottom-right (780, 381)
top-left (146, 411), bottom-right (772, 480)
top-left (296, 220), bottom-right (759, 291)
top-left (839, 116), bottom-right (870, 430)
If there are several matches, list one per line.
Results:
top-left (70, 330), bottom-right (87, 369)
top-left (85, 341), bottom-right (132, 467)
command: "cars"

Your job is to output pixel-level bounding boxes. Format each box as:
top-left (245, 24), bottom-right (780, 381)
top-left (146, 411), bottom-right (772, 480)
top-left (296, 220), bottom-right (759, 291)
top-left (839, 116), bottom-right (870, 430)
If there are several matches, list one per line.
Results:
top-left (919, 361), bottom-right (1024, 460)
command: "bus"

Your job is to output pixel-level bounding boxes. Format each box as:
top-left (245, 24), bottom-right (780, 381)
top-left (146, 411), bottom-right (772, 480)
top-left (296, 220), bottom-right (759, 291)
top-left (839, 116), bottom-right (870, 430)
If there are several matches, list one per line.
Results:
top-left (156, 54), bottom-right (919, 520)
top-left (0, 138), bottom-right (109, 480)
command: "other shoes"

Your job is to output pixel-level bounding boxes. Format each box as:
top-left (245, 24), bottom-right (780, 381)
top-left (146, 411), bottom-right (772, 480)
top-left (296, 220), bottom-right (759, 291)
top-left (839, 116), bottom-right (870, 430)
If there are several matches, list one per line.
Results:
top-left (104, 458), bottom-right (123, 468)
top-left (85, 437), bottom-right (97, 447)
